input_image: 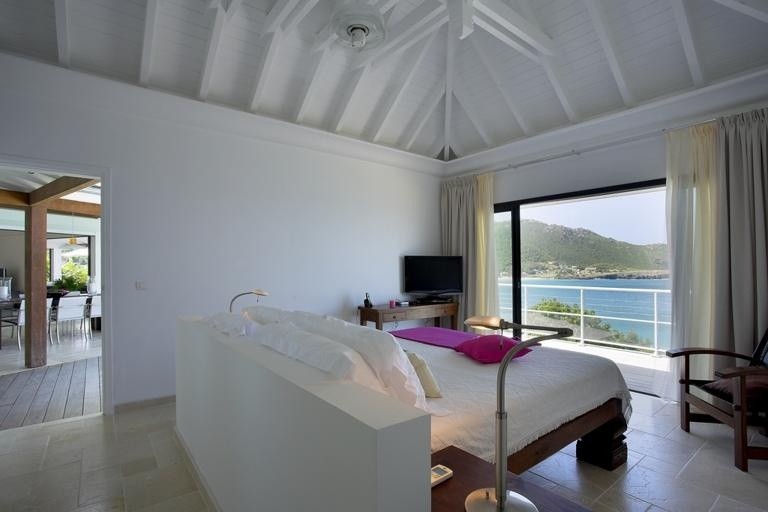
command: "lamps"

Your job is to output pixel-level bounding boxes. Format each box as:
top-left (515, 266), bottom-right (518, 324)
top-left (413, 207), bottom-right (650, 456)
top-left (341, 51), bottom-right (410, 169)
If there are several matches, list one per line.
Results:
top-left (329, 0), bottom-right (387, 52)
top-left (229, 289), bottom-right (269, 313)
top-left (464, 315), bottom-right (574, 510)
top-left (69, 213), bottom-right (77, 245)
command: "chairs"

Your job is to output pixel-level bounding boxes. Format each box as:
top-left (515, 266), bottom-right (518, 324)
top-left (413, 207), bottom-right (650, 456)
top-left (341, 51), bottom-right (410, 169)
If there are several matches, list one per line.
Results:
top-left (0, 291), bottom-right (101, 350)
top-left (666, 323), bottom-right (768, 471)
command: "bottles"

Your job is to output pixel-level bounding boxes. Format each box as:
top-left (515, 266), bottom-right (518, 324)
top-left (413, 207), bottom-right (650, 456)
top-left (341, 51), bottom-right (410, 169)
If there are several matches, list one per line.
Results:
top-left (364, 293), bottom-right (373, 308)
top-left (86, 276), bottom-right (98, 296)
top-left (390, 296), bottom-right (396, 309)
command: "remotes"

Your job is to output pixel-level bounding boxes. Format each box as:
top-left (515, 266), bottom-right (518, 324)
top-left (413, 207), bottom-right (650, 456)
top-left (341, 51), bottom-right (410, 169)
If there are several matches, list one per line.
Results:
top-left (431, 464), bottom-right (453, 488)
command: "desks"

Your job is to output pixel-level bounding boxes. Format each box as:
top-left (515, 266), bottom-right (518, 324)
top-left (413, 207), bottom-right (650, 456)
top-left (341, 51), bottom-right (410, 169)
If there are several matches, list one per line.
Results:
top-left (358, 301), bottom-right (460, 331)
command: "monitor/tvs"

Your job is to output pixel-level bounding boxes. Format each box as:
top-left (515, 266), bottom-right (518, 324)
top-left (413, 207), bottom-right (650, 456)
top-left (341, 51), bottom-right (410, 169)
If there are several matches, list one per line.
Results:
top-left (405, 256), bottom-right (463, 303)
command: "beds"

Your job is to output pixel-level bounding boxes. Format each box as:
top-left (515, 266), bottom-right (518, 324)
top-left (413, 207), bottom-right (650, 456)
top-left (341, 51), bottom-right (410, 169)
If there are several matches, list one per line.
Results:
top-left (177, 307), bottom-right (632, 511)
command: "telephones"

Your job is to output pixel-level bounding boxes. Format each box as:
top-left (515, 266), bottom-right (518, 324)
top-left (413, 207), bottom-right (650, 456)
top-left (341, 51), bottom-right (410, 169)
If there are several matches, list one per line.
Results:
top-left (364, 293), bottom-right (372, 307)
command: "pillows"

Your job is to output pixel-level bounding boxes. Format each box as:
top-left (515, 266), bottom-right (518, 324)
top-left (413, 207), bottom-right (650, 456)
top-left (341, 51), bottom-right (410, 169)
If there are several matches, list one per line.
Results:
top-left (704, 370), bottom-right (768, 404)
top-left (455, 334), bottom-right (532, 364)
top-left (203, 307), bottom-right (451, 416)
